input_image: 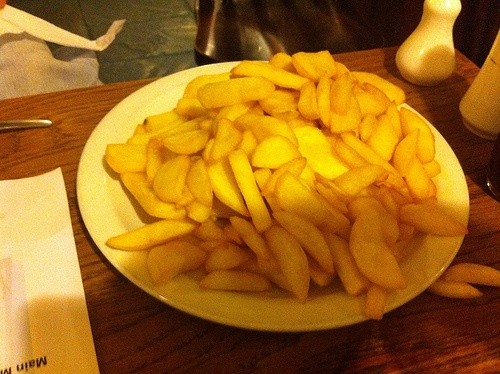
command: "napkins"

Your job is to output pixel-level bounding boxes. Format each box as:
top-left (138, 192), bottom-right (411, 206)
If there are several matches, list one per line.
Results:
top-left (0, 167), bottom-right (99, 374)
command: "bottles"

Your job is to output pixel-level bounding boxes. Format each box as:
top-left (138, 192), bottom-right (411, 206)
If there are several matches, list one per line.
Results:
top-left (459, 28), bottom-right (500, 141)
top-left (396, 0), bottom-right (462, 86)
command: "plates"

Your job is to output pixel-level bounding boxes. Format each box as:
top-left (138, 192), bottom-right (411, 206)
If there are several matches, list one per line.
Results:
top-left (76, 62), bottom-right (470, 333)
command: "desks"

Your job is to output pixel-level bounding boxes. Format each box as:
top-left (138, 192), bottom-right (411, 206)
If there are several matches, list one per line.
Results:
top-left (0, 46), bottom-right (500, 374)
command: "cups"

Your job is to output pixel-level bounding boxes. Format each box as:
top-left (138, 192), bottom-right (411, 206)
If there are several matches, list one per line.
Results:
top-left (487, 132), bottom-right (500, 202)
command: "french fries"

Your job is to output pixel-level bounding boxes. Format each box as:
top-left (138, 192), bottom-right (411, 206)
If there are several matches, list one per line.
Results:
top-left (104, 50), bottom-right (469, 321)
top-left (423, 262), bottom-right (500, 299)
